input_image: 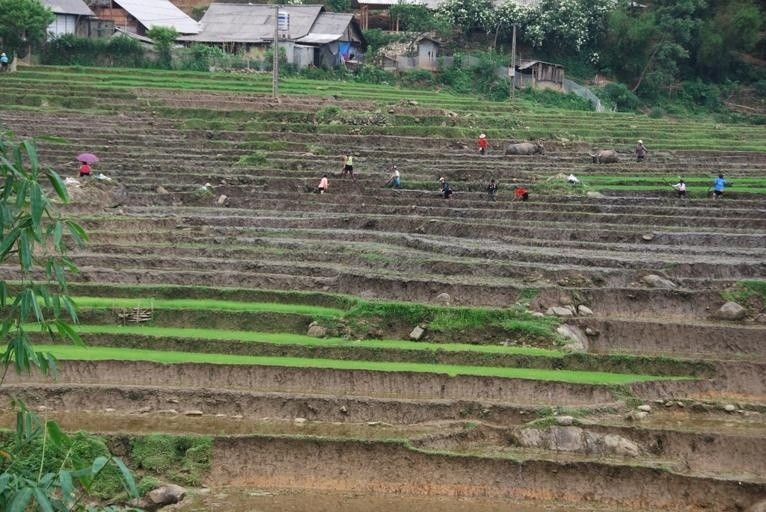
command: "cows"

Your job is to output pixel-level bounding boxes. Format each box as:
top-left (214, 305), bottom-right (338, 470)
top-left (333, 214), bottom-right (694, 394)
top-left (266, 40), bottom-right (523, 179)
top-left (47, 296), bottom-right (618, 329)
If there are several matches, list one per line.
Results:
top-left (545, 172), bottom-right (580, 186)
top-left (505, 141), bottom-right (545, 155)
top-left (588, 150), bottom-right (623, 164)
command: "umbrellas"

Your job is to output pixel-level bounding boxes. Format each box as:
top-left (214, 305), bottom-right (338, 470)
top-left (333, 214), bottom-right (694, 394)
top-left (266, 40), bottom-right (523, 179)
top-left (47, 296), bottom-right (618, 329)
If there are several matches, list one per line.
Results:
top-left (75, 152), bottom-right (99, 163)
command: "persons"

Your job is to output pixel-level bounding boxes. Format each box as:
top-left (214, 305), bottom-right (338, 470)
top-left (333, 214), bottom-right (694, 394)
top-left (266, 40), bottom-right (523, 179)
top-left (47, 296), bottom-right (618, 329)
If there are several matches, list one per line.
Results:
top-left (382, 164), bottom-right (401, 189)
top-left (439, 176), bottom-right (452, 198)
top-left (76, 161), bottom-right (93, 178)
top-left (318, 174), bottom-right (331, 194)
top-left (475, 132), bottom-right (490, 155)
top-left (709, 173), bottom-right (727, 200)
top-left (635, 138), bottom-right (649, 163)
top-left (339, 150), bottom-right (358, 182)
top-left (668, 179), bottom-right (687, 198)
top-left (511, 185), bottom-right (529, 202)
top-left (488, 177), bottom-right (499, 201)
top-left (0, 52), bottom-right (9, 72)
